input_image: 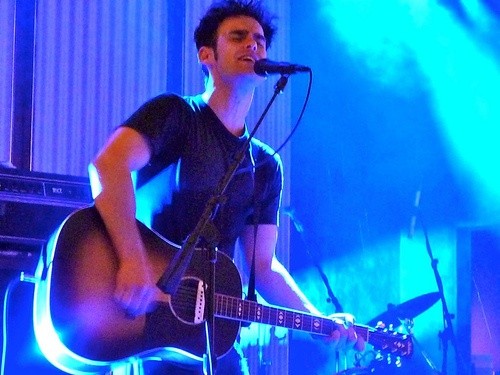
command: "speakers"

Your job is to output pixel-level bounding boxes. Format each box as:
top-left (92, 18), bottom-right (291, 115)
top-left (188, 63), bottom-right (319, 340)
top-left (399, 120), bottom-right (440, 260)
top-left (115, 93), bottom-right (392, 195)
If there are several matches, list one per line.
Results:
top-left (0, 163), bottom-right (95, 248)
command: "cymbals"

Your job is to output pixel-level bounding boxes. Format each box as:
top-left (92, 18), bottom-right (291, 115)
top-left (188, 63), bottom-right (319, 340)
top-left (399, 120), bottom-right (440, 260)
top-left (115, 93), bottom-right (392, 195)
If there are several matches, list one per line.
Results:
top-left (365, 291), bottom-right (442, 329)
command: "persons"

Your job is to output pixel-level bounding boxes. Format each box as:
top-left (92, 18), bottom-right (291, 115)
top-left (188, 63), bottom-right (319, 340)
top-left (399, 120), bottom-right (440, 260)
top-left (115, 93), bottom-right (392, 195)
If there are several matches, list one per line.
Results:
top-left (88, 0), bottom-right (366, 375)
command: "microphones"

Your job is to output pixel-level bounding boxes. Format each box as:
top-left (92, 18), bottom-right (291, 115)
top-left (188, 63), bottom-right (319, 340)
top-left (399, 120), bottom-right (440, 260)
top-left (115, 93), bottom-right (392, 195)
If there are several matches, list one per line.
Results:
top-left (254, 58), bottom-right (310, 78)
top-left (282, 204), bottom-right (305, 234)
top-left (407, 190), bottom-right (422, 239)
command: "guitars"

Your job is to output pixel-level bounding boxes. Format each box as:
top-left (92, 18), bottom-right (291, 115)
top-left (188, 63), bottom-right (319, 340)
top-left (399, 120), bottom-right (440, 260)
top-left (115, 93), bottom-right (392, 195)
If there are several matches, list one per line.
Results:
top-left (29, 204), bottom-right (414, 375)
